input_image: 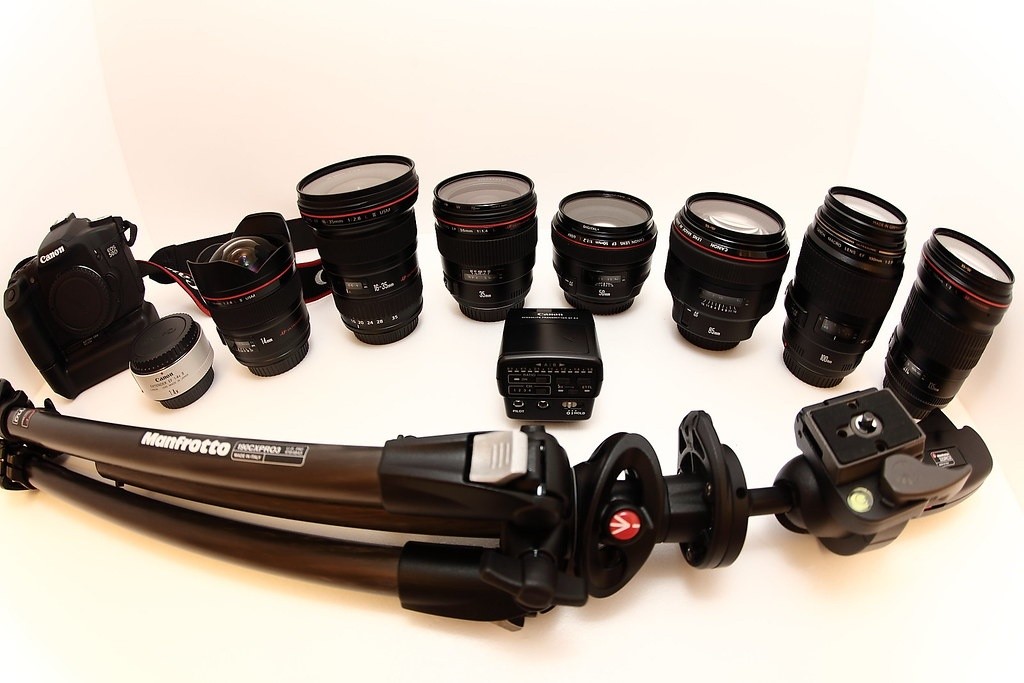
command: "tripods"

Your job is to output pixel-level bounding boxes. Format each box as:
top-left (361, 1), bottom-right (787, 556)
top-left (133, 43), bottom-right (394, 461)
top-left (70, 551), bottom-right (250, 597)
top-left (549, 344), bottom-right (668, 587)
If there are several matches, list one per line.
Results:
top-left (0, 377), bottom-right (991, 630)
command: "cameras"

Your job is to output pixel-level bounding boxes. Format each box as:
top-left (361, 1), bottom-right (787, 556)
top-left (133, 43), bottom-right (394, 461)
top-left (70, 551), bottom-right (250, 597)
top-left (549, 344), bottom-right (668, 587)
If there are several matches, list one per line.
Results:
top-left (3, 212), bottom-right (156, 398)
